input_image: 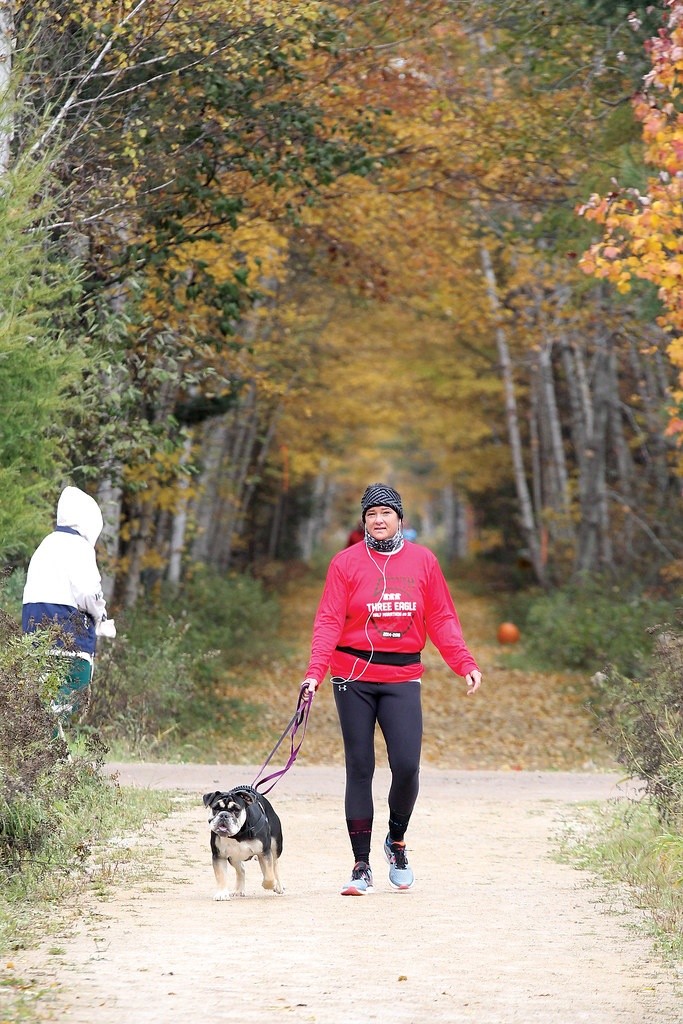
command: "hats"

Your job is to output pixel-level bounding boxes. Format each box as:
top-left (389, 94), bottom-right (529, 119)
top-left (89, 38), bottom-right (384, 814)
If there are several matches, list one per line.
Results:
top-left (361, 482), bottom-right (404, 524)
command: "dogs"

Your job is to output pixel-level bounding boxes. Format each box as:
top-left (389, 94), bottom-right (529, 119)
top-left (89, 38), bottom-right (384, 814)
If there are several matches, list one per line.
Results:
top-left (203, 787), bottom-right (286, 901)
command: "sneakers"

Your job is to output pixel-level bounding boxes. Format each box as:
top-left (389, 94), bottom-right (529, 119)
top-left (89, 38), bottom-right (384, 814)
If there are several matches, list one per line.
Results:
top-left (341, 861), bottom-right (374, 895)
top-left (383, 830), bottom-right (415, 889)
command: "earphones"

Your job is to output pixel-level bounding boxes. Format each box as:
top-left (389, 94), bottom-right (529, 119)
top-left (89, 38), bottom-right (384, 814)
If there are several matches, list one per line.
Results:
top-left (398, 519), bottom-right (401, 529)
top-left (365, 523), bottom-right (367, 529)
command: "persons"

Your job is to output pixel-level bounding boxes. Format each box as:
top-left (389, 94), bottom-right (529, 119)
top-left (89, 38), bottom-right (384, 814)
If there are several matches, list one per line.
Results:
top-left (301, 482), bottom-right (482, 895)
top-left (22, 485), bottom-right (116, 764)
